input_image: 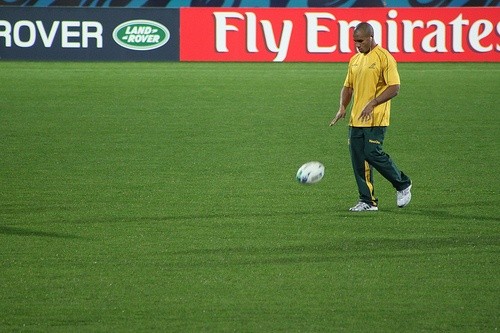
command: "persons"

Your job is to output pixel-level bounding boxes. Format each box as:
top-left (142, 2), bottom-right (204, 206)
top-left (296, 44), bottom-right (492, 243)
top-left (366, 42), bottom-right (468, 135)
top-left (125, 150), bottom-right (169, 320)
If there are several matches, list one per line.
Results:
top-left (329, 19), bottom-right (412, 212)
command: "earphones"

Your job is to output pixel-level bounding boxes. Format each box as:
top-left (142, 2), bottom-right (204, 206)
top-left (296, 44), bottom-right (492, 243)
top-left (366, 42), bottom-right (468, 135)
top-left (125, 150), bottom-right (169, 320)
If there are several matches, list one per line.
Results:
top-left (370, 37), bottom-right (372, 43)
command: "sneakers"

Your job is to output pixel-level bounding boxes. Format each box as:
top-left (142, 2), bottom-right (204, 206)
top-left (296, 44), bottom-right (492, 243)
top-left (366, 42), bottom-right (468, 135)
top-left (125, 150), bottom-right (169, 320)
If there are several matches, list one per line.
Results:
top-left (396, 182), bottom-right (415, 207)
top-left (351, 201), bottom-right (379, 212)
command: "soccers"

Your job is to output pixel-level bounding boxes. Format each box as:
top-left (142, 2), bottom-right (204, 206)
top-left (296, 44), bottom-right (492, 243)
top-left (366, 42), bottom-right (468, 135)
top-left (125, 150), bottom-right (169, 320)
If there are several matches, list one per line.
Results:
top-left (296, 162), bottom-right (325, 184)
